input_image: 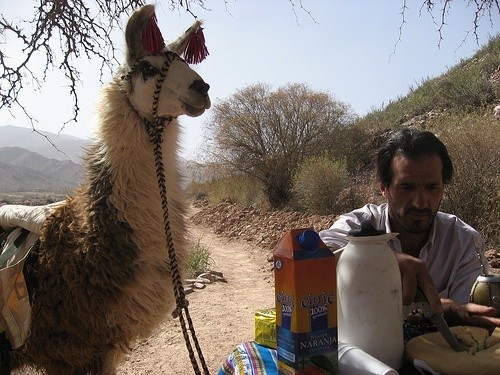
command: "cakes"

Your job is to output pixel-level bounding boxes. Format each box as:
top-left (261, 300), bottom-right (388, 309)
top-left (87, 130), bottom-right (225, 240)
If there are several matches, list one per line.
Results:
top-left (405, 326), bottom-right (500, 375)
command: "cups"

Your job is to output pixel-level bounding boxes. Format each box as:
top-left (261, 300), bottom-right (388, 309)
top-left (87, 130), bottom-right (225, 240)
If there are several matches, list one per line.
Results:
top-left (336, 340), bottom-right (398, 375)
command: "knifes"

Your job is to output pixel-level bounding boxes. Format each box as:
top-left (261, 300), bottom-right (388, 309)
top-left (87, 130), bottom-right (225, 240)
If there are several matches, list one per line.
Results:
top-left (415, 286), bottom-right (462, 350)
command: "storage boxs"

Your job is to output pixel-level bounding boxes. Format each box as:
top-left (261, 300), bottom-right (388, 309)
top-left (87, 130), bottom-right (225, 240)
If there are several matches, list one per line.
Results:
top-left (254, 307), bottom-right (277, 349)
top-left (273, 227), bottom-right (339, 375)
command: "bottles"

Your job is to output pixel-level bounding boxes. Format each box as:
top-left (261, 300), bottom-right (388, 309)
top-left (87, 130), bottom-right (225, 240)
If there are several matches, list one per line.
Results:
top-left (336, 232), bottom-right (404, 367)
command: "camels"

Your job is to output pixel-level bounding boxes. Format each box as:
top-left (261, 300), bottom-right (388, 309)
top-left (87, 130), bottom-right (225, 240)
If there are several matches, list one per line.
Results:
top-left (0, 4), bottom-right (211, 375)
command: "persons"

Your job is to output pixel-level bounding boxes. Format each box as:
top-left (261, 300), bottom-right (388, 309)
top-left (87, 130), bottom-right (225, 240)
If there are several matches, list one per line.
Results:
top-left (318, 129), bottom-right (491, 329)
